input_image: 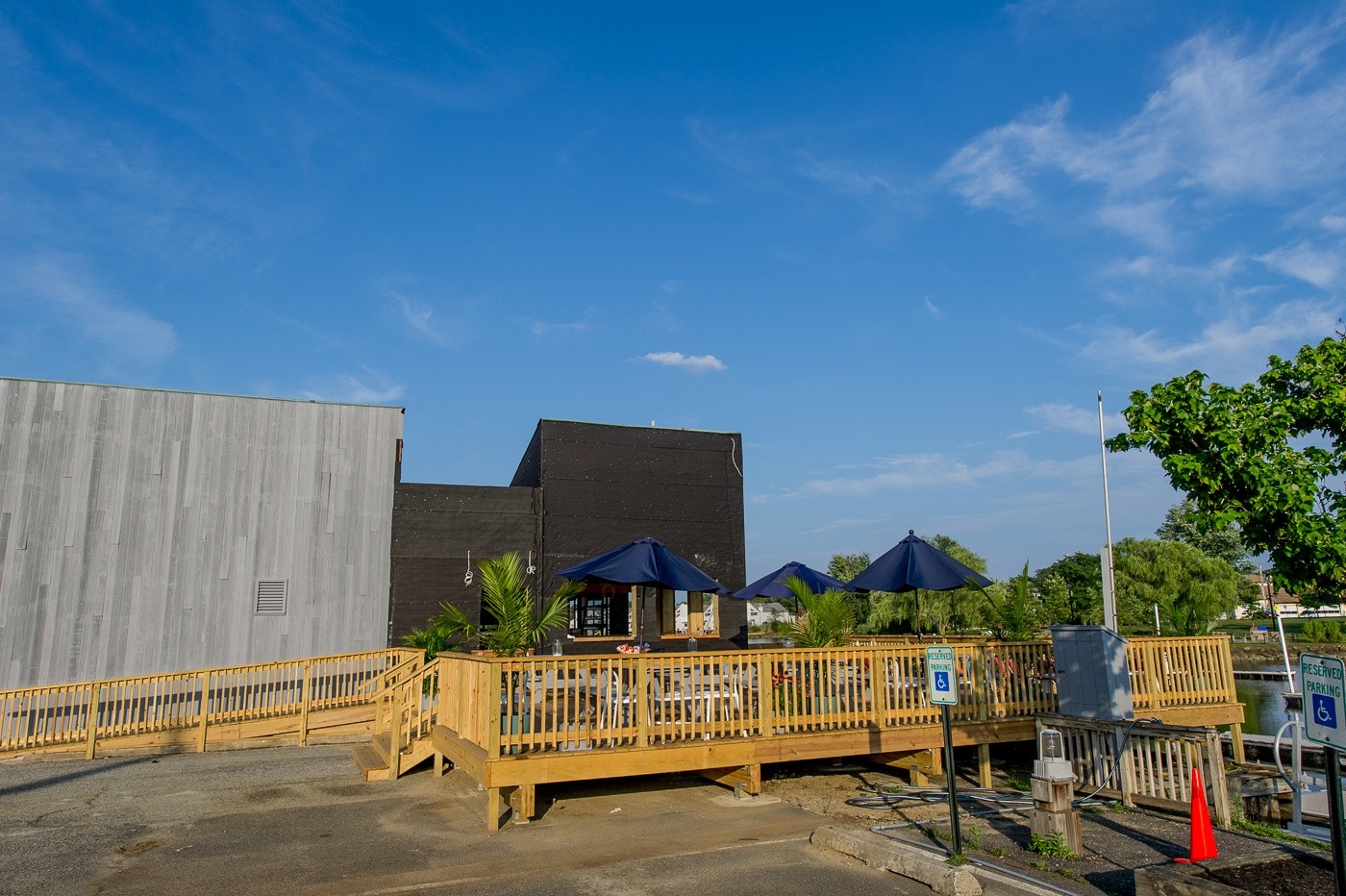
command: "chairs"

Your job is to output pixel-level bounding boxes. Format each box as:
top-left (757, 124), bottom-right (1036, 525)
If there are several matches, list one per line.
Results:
top-left (696, 666), bottom-right (749, 739)
top-left (599, 668), bottom-right (653, 749)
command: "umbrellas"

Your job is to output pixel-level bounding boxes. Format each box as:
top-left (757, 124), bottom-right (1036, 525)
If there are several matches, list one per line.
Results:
top-left (555, 538), bottom-right (735, 655)
top-left (844, 529), bottom-right (995, 644)
top-left (732, 561), bottom-right (847, 623)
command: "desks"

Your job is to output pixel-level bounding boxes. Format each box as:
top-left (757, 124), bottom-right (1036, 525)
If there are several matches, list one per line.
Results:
top-left (646, 688), bottom-right (711, 743)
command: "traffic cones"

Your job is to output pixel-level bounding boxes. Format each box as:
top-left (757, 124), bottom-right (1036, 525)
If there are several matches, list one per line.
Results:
top-left (1171, 768), bottom-right (1218, 864)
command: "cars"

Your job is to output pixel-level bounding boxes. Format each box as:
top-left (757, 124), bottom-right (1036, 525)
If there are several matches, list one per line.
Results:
top-left (1264, 610), bottom-right (1277, 619)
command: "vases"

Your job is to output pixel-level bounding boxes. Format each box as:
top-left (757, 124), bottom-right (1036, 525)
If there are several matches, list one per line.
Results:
top-left (773, 709), bottom-right (786, 735)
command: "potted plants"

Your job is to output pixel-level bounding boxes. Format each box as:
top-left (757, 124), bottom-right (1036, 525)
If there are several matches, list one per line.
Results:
top-left (430, 549), bottom-right (589, 757)
top-left (984, 559), bottom-right (1043, 716)
top-left (770, 576), bottom-right (860, 730)
top-left (396, 616), bottom-right (477, 712)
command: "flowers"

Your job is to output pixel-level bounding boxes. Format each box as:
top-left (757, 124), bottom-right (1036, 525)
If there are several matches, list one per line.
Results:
top-left (751, 671), bottom-right (812, 719)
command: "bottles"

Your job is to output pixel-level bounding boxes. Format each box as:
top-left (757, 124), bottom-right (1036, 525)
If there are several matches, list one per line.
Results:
top-left (552, 639), bottom-right (563, 655)
top-left (687, 636), bottom-right (698, 653)
top-left (782, 637), bottom-right (795, 649)
